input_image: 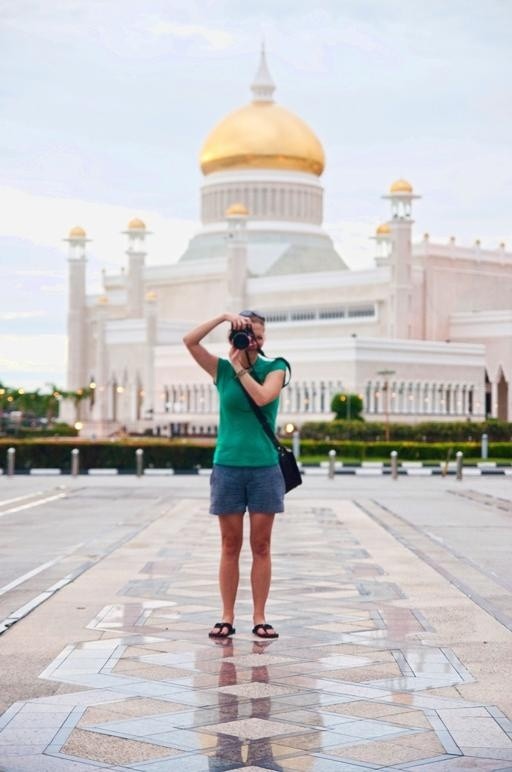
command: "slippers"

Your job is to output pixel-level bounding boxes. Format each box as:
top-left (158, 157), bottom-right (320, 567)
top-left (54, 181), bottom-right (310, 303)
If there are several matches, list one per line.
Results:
top-left (253, 623), bottom-right (279, 638)
top-left (209, 622), bottom-right (235, 638)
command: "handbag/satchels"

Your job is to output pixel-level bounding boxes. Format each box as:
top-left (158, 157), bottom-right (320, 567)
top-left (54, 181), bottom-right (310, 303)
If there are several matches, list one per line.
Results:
top-left (279, 445), bottom-right (302, 494)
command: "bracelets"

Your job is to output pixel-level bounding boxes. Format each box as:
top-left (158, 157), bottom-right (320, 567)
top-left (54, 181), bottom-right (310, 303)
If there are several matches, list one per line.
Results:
top-left (235, 368), bottom-right (249, 379)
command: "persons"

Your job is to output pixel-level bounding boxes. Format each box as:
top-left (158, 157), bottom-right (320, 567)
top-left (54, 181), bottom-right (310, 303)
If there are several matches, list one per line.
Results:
top-left (182, 309), bottom-right (288, 638)
top-left (206, 639), bottom-right (285, 772)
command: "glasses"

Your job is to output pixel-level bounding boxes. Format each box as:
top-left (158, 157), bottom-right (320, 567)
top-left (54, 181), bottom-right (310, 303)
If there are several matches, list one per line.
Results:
top-left (241, 310), bottom-right (265, 321)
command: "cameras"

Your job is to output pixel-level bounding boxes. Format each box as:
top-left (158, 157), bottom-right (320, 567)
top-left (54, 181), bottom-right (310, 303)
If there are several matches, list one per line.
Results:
top-left (229, 322), bottom-right (255, 350)
